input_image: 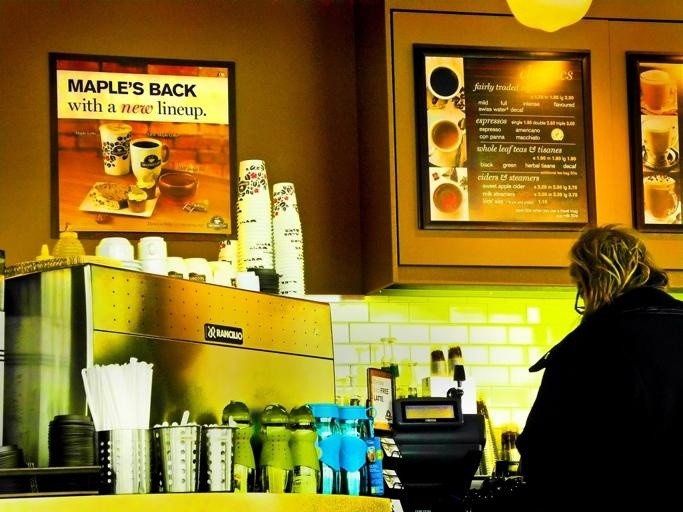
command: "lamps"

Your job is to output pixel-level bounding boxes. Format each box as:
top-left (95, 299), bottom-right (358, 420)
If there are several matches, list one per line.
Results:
top-left (501, 0), bottom-right (597, 36)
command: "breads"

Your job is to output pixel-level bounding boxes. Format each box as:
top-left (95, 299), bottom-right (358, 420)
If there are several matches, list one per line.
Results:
top-left (90, 182), bottom-right (129, 210)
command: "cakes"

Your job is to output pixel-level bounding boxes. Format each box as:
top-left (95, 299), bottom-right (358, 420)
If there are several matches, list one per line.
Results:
top-left (136, 172), bottom-right (156, 200)
top-left (127, 184), bottom-right (147, 213)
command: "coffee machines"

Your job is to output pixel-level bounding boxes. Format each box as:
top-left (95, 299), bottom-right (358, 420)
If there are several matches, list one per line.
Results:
top-left (3, 267), bottom-right (336, 491)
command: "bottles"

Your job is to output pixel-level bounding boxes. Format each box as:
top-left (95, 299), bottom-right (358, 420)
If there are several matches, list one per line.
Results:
top-left (35, 222), bottom-right (85, 261)
top-left (220, 398), bottom-right (384, 495)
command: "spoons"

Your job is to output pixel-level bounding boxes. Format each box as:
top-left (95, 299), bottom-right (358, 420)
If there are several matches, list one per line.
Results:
top-left (453, 120), bottom-right (465, 167)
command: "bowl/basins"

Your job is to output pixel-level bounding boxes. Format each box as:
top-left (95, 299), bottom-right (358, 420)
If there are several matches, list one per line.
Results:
top-left (158, 172), bottom-right (198, 199)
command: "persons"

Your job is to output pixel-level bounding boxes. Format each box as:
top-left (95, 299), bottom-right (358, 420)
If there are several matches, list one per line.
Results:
top-left (511, 220), bottom-right (682, 511)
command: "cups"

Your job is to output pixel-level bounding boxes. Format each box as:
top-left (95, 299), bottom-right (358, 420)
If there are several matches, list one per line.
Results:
top-left (128, 137), bottom-right (170, 187)
top-left (429, 346), bottom-right (461, 379)
top-left (427, 67), bottom-right (460, 100)
top-left (644, 176), bottom-right (680, 222)
top-left (639, 69), bottom-right (676, 113)
top-left (99, 124), bottom-right (130, 174)
top-left (433, 182), bottom-right (463, 216)
top-left (646, 121), bottom-right (679, 164)
top-left (475, 399), bottom-right (520, 475)
top-left (88, 238), bottom-right (261, 292)
top-left (431, 121), bottom-right (462, 152)
top-left (237, 158), bottom-right (305, 299)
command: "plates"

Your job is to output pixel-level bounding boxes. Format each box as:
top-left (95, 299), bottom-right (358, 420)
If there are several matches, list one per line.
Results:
top-left (77, 182), bottom-right (159, 217)
top-left (641, 146), bottom-right (679, 174)
top-left (428, 107), bottom-right (465, 166)
